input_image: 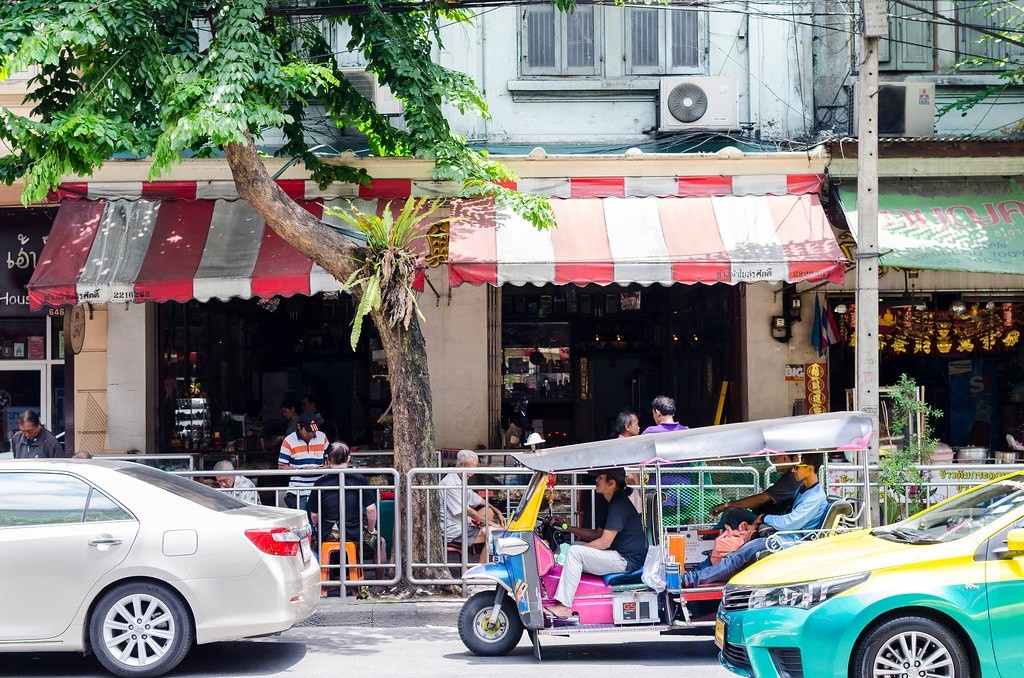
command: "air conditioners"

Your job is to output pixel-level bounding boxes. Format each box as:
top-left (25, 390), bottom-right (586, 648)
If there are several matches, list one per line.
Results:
top-left (656, 75), bottom-right (740, 130)
top-left (848, 81), bottom-right (935, 137)
top-left (324, 67), bottom-right (404, 116)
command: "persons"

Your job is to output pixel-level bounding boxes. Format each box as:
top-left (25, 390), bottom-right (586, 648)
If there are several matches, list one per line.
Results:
top-left (642, 396), bottom-right (693, 533)
top-left (12, 410), bottom-right (92, 459)
top-left (682, 454), bottom-right (827, 588)
top-left (541, 466), bottom-right (645, 622)
top-left (308, 443), bottom-right (387, 580)
top-left (438, 450), bottom-right (506, 564)
top-left (214, 460), bottom-right (261, 504)
top-left (272, 398), bottom-right (328, 444)
top-left (618, 414), bottom-right (649, 514)
top-left (278, 414), bottom-right (331, 510)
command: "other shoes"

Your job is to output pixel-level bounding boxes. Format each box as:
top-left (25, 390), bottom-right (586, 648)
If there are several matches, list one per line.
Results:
top-left (681, 572), bottom-right (694, 589)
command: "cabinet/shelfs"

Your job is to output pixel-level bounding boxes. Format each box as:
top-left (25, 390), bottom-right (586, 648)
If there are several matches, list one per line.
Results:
top-left (174, 398), bottom-right (212, 447)
top-left (501, 317), bottom-right (582, 406)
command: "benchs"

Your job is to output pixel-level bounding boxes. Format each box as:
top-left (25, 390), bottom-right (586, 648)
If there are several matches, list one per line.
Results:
top-left (752, 496), bottom-right (866, 564)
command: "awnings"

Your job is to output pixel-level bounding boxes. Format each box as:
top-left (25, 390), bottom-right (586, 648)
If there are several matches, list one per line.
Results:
top-left (838, 178), bottom-right (1024, 275)
top-left (28, 195), bottom-right (429, 311)
top-left (449, 193), bottom-right (845, 286)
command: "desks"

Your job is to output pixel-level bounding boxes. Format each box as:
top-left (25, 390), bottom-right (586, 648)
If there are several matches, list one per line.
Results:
top-left (362, 498), bottom-right (394, 558)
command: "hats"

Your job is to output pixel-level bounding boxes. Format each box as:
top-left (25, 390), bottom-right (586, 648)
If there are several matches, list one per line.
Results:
top-left (588, 467), bottom-right (625, 480)
top-left (299, 411), bottom-right (320, 432)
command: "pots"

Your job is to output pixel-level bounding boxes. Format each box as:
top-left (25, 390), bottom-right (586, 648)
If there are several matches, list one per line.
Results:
top-left (954, 445), bottom-right (990, 465)
top-left (994, 452), bottom-right (1021, 464)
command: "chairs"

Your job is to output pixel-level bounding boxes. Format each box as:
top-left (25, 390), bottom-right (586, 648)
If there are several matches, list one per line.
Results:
top-left (441, 542), bottom-right (462, 576)
top-left (603, 492), bottom-right (667, 585)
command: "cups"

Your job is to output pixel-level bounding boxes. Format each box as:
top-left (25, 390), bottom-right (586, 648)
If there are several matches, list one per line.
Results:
top-left (666, 563), bottom-right (681, 594)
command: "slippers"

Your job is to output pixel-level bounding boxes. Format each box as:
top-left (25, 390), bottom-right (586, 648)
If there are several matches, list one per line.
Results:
top-left (542, 604), bottom-right (572, 620)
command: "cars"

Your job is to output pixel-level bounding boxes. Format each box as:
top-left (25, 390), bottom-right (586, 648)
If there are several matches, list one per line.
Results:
top-left (716, 468), bottom-right (1024, 678)
top-left (0, 460), bottom-right (323, 678)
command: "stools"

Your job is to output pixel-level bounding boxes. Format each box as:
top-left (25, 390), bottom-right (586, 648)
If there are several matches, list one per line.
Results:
top-left (319, 541), bottom-right (366, 596)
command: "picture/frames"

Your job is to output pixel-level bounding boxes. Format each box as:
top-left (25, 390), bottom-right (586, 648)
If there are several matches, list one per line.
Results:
top-left (503, 288), bottom-right (667, 325)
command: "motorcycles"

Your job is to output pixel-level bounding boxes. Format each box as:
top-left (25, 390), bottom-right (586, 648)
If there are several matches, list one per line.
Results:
top-left (457, 411), bottom-right (874, 658)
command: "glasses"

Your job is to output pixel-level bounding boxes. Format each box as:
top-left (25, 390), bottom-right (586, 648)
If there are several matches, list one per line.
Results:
top-left (793, 463), bottom-right (813, 470)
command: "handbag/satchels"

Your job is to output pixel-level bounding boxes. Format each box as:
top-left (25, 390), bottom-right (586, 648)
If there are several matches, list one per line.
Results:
top-left (641, 537), bottom-right (669, 593)
top-left (710, 522), bottom-right (756, 566)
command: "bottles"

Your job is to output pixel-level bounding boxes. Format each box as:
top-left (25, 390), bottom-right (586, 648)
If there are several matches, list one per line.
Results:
top-left (539, 353), bottom-right (571, 398)
